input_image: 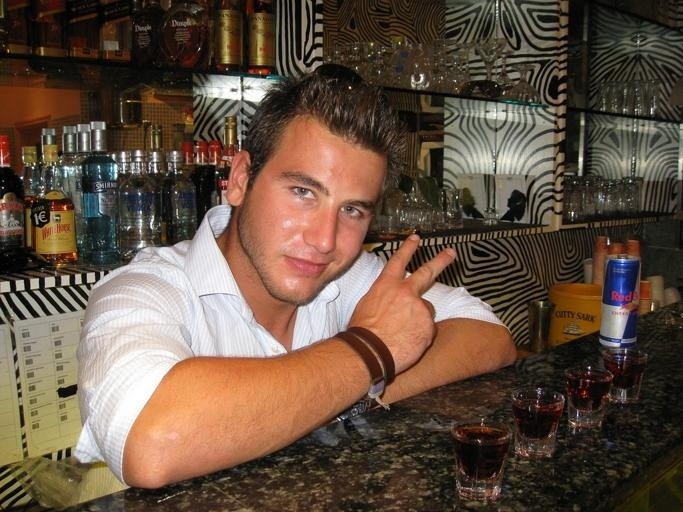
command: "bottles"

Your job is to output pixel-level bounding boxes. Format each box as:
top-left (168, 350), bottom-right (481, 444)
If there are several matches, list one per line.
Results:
top-left (1, 112), bottom-right (239, 270)
top-left (0, 2), bottom-right (277, 76)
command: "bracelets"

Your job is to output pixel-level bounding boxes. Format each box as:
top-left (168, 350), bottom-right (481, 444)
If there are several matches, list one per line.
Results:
top-left (333, 328), bottom-right (385, 408)
top-left (345, 326), bottom-right (395, 387)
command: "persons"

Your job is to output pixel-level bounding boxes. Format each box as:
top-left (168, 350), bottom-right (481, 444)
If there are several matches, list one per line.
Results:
top-left (457, 187), bottom-right (486, 219)
top-left (70, 65), bottom-right (521, 507)
top-left (500, 188), bottom-right (528, 221)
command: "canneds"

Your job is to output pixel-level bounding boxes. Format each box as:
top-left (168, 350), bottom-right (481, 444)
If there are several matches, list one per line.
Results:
top-left (597, 253), bottom-right (643, 350)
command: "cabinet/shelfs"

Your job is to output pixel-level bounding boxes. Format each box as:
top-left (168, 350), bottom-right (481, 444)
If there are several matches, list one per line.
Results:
top-left (0, 43), bottom-right (681, 295)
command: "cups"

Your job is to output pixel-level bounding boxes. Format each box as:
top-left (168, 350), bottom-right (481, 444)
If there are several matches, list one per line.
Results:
top-left (369, 182), bottom-right (464, 238)
top-left (482, 207), bottom-right (502, 227)
top-left (524, 296), bottom-right (554, 356)
top-left (555, 169), bottom-right (644, 226)
top-left (327, 36), bottom-right (433, 89)
top-left (449, 420), bottom-right (513, 510)
top-left (562, 362), bottom-right (611, 434)
top-left (583, 234), bottom-right (677, 313)
top-left (595, 76), bottom-right (664, 121)
top-left (510, 388), bottom-right (566, 459)
top-left (601, 345), bottom-right (652, 414)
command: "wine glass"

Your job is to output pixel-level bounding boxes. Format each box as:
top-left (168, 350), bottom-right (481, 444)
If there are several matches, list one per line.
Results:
top-left (496, 51), bottom-right (514, 99)
top-left (435, 41), bottom-right (472, 94)
top-left (474, 39), bottom-right (506, 82)
top-left (509, 66), bottom-right (541, 109)
